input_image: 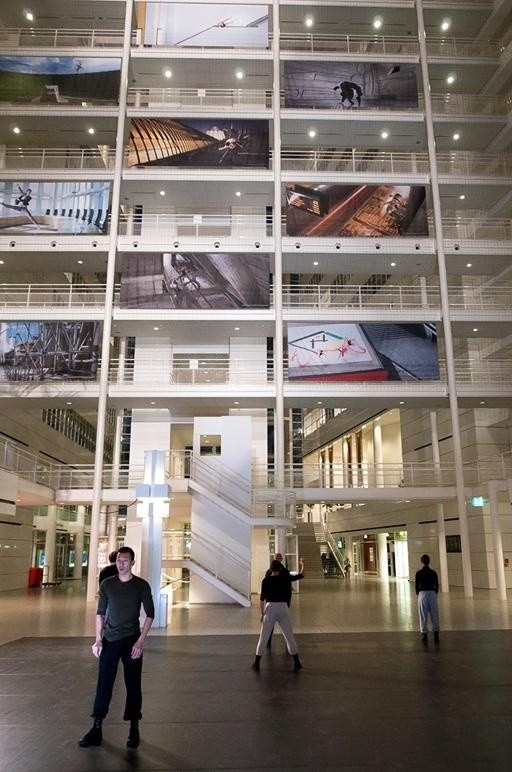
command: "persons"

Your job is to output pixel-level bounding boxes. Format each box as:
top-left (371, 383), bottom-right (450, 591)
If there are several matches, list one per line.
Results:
top-left (98, 550), bottom-right (119, 586)
top-left (414, 554), bottom-right (440, 646)
top-left (252, 560), bottom-right (303, 674)
top-left (264, 552), bottom-right (305, 649)
top-left (79, 547), bottom-right (155, 750)
top-left (1, 182), bottom-right (42, 231)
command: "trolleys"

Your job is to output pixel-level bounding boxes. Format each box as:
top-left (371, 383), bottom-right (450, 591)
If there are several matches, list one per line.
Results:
top-left (42, 564), bottom-right (65, 589)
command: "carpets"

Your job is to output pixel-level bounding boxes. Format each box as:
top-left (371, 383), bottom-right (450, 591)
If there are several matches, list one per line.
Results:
top-left (0, 628), bottom-right (511, 772)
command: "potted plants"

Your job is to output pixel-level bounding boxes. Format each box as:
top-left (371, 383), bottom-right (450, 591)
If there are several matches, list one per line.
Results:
top-left (343, 553), bottom-right (352, 581)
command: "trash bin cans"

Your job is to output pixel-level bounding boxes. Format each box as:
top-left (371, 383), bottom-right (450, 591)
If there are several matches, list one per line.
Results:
top-left (28, 567), bottom-right (43, 589)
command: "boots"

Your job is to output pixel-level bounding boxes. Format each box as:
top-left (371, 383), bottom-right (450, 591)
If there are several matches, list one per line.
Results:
top-left (78, 727), bottom-right (102, 748)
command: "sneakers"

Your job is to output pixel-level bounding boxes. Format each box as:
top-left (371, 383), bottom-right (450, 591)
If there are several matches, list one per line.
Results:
top-left (125, 727), bottom-right (141, 749)
top-left (250, 662), bottom-right (261, 674)
top-left (293, 661), bottom-right (303, 672)
top-left (421, 638), bottom-right (440, 645)
